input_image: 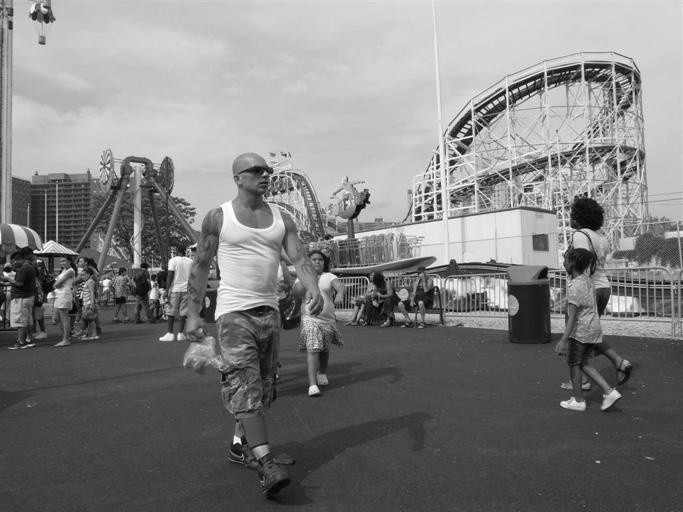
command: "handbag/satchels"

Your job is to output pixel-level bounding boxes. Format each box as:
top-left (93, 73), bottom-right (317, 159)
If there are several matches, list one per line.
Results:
top-left (41, 267), bottom-right (54, 292)
top-left (136, 271), bottom-right (149, 297)
top-left (278, 284), bottom-right (302, 330)
top-left (68, 301), bottom-right (77, 314)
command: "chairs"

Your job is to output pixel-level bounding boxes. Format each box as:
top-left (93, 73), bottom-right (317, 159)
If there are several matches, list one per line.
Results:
top-left (392, 286), bottom-right (444, 327)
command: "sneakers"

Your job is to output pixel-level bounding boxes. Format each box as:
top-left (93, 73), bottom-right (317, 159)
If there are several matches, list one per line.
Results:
top-left (25, 340), bottom-right (35, 348)
top-left (259, 453), bottom-right (291, 499)
top-left (601, 388), bottom-right (622, 411)
top-left (560, 397), bottom-right (586, 411)
top-left (8, 338), bottom-right (28, 350)
top-left (177, 333), bottom-right (186, 340)
top-left (318, 372), bottom-right (327, 385)
top-left (229, 436), bottom-right (260, 472)
top-left (616, 360), bottom-right (632, 385)
top-left (159, 333), bottom-right (174, 342)
top-left (308, 386), bottom-right (319, 396)
top-left (560, 382), bottom-right (592, 391)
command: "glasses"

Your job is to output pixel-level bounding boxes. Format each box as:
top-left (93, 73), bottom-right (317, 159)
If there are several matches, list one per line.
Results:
top-left (237, 166), bottom-right (273, 176)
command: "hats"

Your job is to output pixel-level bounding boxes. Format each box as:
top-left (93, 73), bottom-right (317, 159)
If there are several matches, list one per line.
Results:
top-left (4, 263), bottom-right (13, 269)
top-left (417, 266), bottom-right (425, 272)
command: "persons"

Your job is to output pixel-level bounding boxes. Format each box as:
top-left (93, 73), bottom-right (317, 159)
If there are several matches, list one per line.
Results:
top-left (54, 257), bottom-right (75, 347)
top-left (554, 196), bottom-right (631, 392)
top-left (157, 265), bottom-right (168, 320)
top-left (398, 267), bottom-right (433, 329)
top-left (113, 267), bottom-right (130, 322)
top-left (33, 259), bottom-right (48, 339)
top-left (74, 256), bottom-right (100, 337)
top-left (9, 252), bottom-right (36, 349)
top-left (183, 154), bottom-right (324, 499)
top-left (158, 244), bottom-right (193, 342)
top-left (150, 273), bottom-right (160, 321)
top-left (556, 249), bottom-right (622, 412)
top-left (102, 274), bottom-right (111, 307)
top-left (134, 264), bottom-right (159, 323)
top-left (296, 251), bottom-right (347, 396)
top-left (345, 272), bottom-right (398, 328)
top-left (1, 263), bottom-right (17, 330)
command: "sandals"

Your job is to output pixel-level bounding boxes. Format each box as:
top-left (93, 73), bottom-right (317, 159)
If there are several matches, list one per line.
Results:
top-left (418, 321), bottom-right (425, 328)
top-left (402, 321), bottom-right (414, 328)
top-left (53, 340), bottom-right (71, 347)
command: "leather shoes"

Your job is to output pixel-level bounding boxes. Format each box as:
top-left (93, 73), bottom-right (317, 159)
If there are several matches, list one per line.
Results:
top-left (71, 330), bottom-right (99, 341)
